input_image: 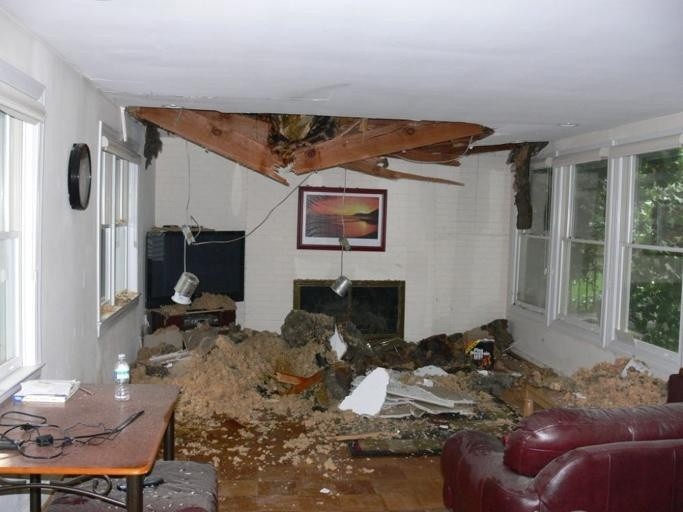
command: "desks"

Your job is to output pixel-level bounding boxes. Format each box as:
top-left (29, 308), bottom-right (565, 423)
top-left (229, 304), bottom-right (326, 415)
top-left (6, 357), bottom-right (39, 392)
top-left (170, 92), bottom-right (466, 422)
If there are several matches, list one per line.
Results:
top-left (0, 379), bottom-right (180, 511)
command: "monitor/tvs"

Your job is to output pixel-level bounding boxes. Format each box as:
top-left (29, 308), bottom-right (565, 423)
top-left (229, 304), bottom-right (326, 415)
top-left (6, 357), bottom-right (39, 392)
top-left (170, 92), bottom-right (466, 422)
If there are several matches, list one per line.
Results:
top-left (145, 229), bottom-right (246, 309)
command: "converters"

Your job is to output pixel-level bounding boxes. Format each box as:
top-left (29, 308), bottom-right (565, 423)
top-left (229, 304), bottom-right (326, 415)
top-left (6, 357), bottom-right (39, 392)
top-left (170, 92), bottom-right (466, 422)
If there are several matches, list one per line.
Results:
top-left (36, 433), bottom-right (54, 446)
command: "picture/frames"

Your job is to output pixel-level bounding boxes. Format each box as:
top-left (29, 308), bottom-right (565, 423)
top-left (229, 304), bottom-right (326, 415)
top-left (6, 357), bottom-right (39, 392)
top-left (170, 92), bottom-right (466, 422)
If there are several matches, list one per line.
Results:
top-left (296, 185), bottom-right (387, 253)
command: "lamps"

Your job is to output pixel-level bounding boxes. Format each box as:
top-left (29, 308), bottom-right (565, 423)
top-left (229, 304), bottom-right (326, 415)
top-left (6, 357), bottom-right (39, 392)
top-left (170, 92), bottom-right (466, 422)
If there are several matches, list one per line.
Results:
top-left (330, 165), bottom-right (353, 300)
top-left (170, 239), bottom-right (199, 307)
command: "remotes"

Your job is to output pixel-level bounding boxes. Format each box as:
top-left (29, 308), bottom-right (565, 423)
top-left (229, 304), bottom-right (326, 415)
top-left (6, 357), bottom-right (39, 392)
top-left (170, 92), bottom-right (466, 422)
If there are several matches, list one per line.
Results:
top-left (118, 477), bottom-right (166, 492)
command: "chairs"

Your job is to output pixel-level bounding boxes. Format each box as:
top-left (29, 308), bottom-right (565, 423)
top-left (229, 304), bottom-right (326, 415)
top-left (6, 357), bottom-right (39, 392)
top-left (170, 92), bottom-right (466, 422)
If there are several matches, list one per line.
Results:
top-left (440, 402), bottom-right (681, 512)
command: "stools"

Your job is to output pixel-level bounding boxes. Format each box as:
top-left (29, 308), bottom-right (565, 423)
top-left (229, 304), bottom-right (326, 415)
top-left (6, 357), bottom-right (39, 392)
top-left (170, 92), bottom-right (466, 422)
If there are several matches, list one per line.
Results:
top-left (41, 461), bottom-right (218, 511)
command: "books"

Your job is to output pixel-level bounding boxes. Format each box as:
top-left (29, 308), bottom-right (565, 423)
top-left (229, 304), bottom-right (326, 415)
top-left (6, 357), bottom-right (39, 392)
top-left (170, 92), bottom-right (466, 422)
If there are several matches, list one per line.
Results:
top-left (21, 380), bottom-right (81, 403)
top-left (14, 379), bottom-right (72, 397)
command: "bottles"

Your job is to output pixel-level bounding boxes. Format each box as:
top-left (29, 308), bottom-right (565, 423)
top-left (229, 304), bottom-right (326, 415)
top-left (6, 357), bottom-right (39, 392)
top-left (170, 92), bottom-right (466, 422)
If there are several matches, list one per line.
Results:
top-left (112, 352), bottom-right (132, 401)
